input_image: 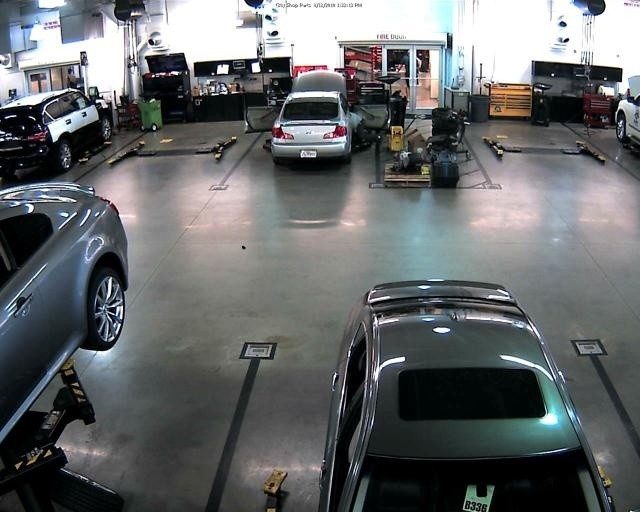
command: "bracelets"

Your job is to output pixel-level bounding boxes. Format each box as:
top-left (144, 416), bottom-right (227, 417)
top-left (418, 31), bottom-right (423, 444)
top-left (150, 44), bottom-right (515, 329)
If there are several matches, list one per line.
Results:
top-left (397, 68), bottom-right (400, 70)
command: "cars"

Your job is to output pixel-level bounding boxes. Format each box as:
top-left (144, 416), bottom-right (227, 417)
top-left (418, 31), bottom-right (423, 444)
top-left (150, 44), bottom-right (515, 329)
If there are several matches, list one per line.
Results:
top-left (315, 279), bottom-right (615, 512)
top-left (246, 71), bottom-right (390, 166)
top-left (615, 76), bottom-right (639, 154)
top-left (0, 183), bottom-right (130, 442)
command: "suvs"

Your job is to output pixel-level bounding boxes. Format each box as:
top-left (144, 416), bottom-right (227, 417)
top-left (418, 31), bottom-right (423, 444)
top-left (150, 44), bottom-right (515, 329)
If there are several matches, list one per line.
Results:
top-left (1, 89), bottom-right (111, 172)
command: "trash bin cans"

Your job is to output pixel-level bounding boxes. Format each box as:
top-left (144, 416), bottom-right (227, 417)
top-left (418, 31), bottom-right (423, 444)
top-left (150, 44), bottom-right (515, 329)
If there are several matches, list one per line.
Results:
top-left (532, 83), bottom-right (553, 126)
top-left (137, 100), bottom-right (162, 132)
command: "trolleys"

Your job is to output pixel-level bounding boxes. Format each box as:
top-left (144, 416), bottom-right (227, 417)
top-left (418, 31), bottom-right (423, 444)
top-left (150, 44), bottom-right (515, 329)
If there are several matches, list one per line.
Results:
top-left (115, 92), bottom-right (141, 131)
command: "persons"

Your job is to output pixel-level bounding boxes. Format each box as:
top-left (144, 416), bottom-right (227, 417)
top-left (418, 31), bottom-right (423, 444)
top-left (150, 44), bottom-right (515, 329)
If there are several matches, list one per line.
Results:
top-left (66, 69), bottom-right (77, 88)
top-left (396, 50), bottom-right (421, 98)
